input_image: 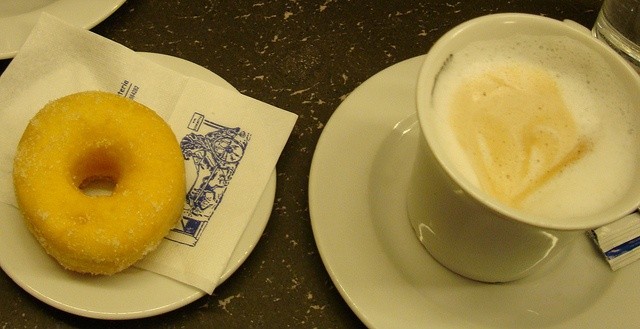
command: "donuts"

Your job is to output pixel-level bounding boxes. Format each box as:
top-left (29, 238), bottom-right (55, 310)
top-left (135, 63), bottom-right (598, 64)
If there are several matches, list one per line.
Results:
top-left (13, 90), bottom-right (186, 275)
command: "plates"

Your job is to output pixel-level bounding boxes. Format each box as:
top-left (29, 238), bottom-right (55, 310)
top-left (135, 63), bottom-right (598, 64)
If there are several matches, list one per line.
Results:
top-left (308, 54), bottom-right (640, 329)
top-left (1, 0), bottom-right (126, 59)
top-left (0, 52), bottom-right (277, 320)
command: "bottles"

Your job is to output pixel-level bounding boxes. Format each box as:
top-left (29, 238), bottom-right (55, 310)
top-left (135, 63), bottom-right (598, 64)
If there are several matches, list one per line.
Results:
top-left (589, 0), bottom-right (640, 77)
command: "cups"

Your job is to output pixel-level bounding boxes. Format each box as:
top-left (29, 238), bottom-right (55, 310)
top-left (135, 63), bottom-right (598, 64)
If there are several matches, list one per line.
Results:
top-left (407, 13), bottom-right (640, 282)
